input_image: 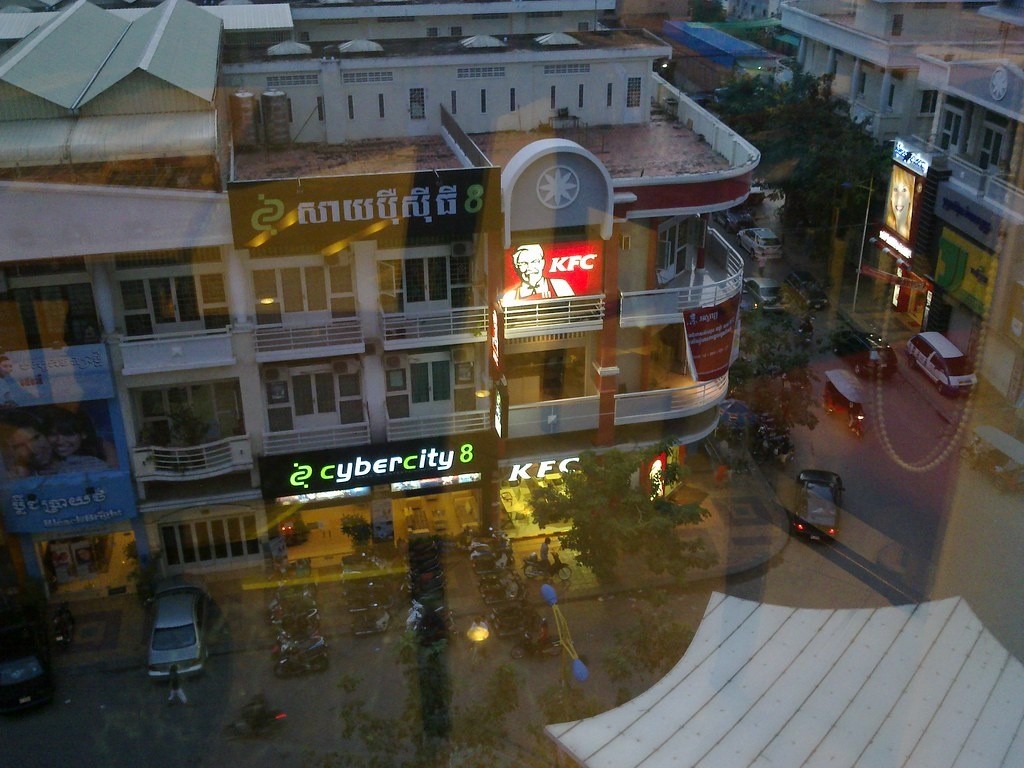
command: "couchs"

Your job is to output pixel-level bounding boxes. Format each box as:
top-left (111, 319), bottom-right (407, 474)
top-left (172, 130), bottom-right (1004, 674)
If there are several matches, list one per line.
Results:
top-left (453, 496), bottom-right (480, 530)
top-left (404, 507), bottom-right (430, 534)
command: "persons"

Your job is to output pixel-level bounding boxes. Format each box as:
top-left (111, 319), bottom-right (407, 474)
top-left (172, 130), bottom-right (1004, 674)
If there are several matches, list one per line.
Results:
top-left (799, 318), bottom-right (813, 334)
top-left (534, 618), bottom-right (549, 662)
top-left (885, 167), bottom-right (914, 240)
top-left (778, 434), bottom-right (789, 467)
top-left (758, 253), bottom-right (767, 278)
top-left (0, 410), bottom-right (63, 478)
top-left (471, 617), bottom-right (489, 629)
top-left (0, 355), bottom-right (13, 378)
top-left (733, 433), bottom-right (749, 474)
top-left (848, 403), bottom-right (864, 431)
top-left (234, 692), bottom-right (268, 737)
top-left (167, 664), bottom-right (192, 706)
top-left (540, 538), bottom-right (550, 580)
top-left (42, 407), bottom-right (117, 474)
top-left (53, 606), bottom-right (74, 642)
top-left (752, 416), bottom-right (770, 454)
top-left (298, 620), bottom-right (313, 671)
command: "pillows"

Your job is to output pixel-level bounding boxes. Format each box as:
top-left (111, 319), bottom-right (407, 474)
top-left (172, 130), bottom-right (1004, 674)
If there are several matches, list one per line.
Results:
top-left (464, 501), bottom-right (472, 514)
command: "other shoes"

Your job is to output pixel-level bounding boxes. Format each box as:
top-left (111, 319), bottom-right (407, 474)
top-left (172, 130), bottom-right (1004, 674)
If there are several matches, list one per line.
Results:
top-left (184, 702), bottom-right (192, 707)
top-left (168, 700), bottom-right (175, 706)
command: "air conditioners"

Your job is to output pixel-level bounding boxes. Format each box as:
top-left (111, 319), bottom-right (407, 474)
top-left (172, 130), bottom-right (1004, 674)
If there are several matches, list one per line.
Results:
top-left (619, 233), bottom-right (630, 251)
top-left (452, 237), bottom-right (472, 257)
top-left (332, 357), bottom-right (358, 375)
top-left (262, 365), bottom-right (290, 384)
top-left (323, 248), bottom-right (348, 267)
top-left (450, 344), bottom-right (474, 364)
top-left (384, 353), bottom-right (408, 371)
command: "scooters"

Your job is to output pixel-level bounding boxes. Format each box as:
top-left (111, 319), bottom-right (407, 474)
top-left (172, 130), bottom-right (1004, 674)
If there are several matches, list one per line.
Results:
top-left (521, 552), bottom-right (573, 583)
top-left (340, 550), bottom-right (396, 637)
top-left (226, 708), bottom-right (287, 737)
top-left (404, 539), bottom-right (456, 640)
top-left (463, 528), bottom-right (540, 638)
top-left (268, 557), bottom-right (331, 679)
top-left (750, 411), bottom-right (793, 455)
top-left (510, 633), bottom-right (562, 659)
top-left (824, 369), bottom-right (868, 437)
top-left (958, 424), bottom-right (1024, 493)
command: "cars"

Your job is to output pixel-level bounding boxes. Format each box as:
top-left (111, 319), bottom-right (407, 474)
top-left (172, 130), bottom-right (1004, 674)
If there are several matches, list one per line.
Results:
top-left (0, 621), bottom-right (47, 712)
top-left (906, 332), bottom-right (978, 397)
top-left (147, 585), bottom-right (209, 682)
top-left (744, 277), bottom-right (790, 315)
top-left (786, 270), bottom-right (827, 311)
top-left (793, 468), bottom-right (846, 541)
top-left (836, 331), bottom-right (899, 381)
top-left (738, 228), bottom-right (783, 266)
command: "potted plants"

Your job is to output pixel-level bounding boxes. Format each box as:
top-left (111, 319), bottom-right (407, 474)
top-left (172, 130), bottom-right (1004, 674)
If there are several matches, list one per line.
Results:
top-left (124, 540), bottom-right (140, 573)
top-left (342, 514), bottom-right (371, 549)
